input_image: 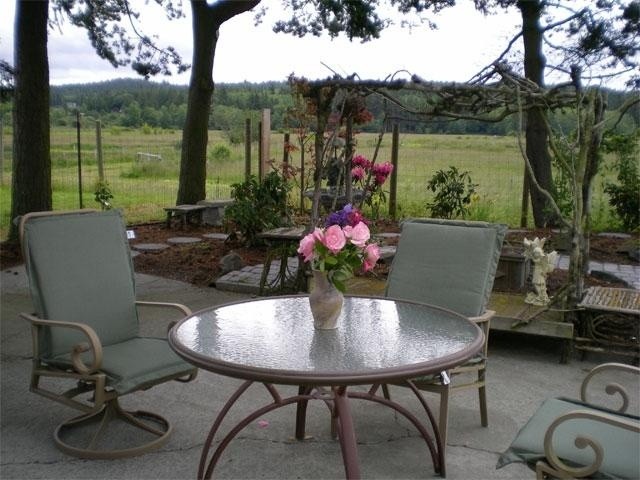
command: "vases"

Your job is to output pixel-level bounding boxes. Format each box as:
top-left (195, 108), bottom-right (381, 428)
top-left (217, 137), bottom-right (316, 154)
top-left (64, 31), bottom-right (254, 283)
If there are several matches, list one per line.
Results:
top-left (309, 269), bottom-right (343, 330)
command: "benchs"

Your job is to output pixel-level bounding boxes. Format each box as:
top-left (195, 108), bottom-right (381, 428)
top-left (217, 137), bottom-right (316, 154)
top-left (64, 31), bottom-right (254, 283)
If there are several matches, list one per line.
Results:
top-left (201, 199), bottom-right (233, 226)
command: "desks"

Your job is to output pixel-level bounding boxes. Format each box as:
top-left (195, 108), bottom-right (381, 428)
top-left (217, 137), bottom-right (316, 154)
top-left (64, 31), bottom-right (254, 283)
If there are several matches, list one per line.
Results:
top-left (168, 294), bottom-right (485, 479)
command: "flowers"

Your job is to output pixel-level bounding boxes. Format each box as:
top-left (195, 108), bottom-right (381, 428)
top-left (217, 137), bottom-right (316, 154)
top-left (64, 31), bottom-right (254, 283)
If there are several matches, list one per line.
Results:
top-left (296, 202), bottom-right (379, 282)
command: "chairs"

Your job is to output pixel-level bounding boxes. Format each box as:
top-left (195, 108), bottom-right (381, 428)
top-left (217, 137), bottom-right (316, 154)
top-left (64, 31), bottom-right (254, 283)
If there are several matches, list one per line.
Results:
top-left (19, 208), bottom-right (197, 459)
top-left (386, 218), bottom-right (506, 447)
top-left (495, 362), bottom-right (640, 480)
top-left (576, 284), bottom-right (640, 348)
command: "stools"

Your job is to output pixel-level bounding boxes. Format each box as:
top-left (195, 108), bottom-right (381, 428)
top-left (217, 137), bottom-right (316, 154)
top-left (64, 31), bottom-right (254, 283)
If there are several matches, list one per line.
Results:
top-left (165, 205), bottom-right (205, 231)
